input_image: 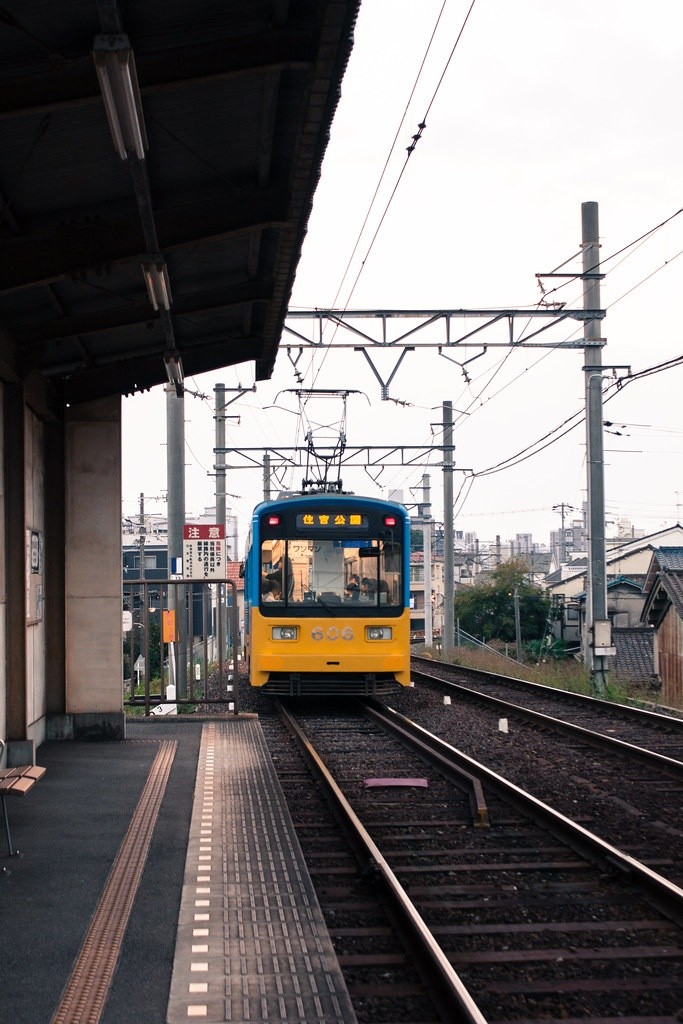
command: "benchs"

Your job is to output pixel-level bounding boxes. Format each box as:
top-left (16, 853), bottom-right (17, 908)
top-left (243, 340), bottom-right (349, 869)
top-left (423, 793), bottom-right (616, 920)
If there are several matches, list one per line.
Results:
top-left (0, 739), bottom-right (47, 860)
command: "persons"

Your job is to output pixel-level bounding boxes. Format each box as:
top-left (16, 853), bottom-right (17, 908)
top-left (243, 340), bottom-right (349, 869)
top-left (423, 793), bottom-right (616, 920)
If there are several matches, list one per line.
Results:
top-left (342, 575), bottom-right (391, 603)
top-left (262, 557), bottom-right (295, 601)
top-left (261, 579), bottom-right (282, 601)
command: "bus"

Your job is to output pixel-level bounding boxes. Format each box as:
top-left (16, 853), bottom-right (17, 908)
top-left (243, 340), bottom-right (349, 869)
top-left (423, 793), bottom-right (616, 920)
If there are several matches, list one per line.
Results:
top-left (239, 492), bottom-right (413, 696)
